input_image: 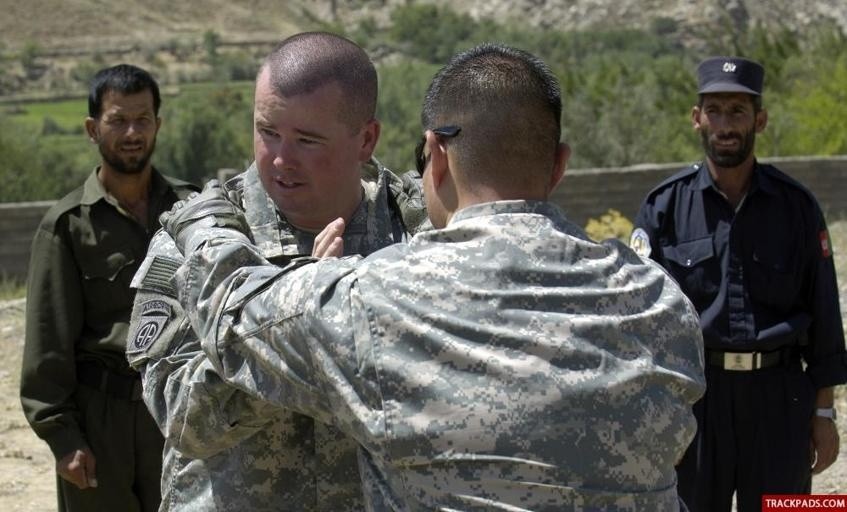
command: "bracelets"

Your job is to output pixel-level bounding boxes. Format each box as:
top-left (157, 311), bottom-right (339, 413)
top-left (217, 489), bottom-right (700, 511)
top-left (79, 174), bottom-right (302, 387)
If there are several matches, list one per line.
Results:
top-left (815, 406), bottom-right (838, 419)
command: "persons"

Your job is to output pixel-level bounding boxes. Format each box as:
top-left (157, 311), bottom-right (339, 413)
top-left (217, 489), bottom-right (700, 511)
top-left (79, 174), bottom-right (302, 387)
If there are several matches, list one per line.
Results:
top-left (630, 58), bottom-right (847, 512)
top-left (18, 64), bottom-right (203, 512)
top-left (156, 44), bottom-right (708, 512)
top-left (123, 31), bottom-right (415, 512)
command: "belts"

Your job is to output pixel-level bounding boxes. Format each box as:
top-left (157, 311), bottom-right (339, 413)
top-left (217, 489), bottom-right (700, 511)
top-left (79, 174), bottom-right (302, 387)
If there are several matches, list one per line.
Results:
top-left (705, 348), bottom-right (795, 373)
top-left (74, 365), bottom-right (143, 403)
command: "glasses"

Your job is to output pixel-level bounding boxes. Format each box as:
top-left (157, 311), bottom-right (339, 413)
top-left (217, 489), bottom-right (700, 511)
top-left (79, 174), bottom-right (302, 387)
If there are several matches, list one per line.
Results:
top-left (416, 127), bottom-right (461, 173)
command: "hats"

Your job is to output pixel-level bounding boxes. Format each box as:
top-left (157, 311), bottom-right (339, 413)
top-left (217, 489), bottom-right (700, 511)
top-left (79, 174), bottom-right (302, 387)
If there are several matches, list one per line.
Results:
top-left (696, 56), bottom-right (765, 97)
